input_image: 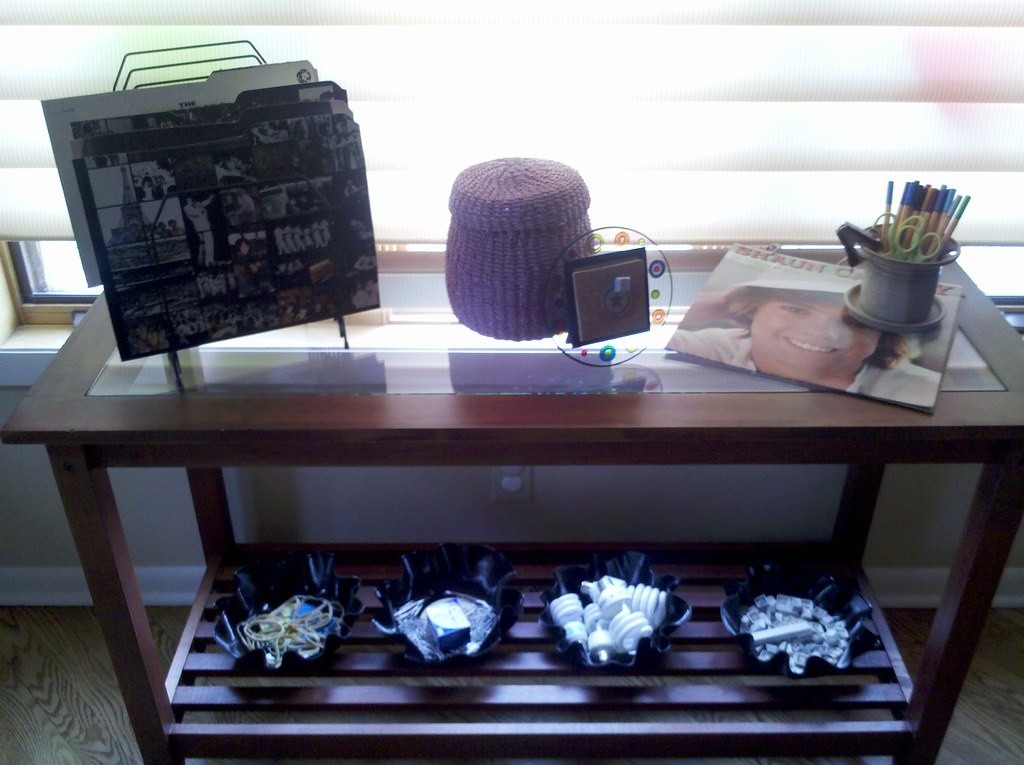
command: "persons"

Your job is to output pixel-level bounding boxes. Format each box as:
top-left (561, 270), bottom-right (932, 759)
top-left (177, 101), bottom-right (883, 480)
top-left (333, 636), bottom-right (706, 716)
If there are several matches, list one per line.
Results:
top-left (77, 89), bottom-right (380, 358)
top-left (667, 277), bottom-right (941, 408)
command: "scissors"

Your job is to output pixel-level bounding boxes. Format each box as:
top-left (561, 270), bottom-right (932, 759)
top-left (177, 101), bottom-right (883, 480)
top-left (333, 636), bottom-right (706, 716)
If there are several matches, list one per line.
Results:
top-left (872, 212), bottom-right (926, 260)
top-left (895, 225), bottom-right (941, 262)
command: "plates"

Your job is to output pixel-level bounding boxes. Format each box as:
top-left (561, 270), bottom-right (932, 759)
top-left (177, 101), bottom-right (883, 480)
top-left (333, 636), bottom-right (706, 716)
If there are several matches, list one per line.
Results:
top-left (719, 559), bottom-right (880, 679)
top-left (213, 550), bottom-right (363, 670)
top-left (373, 541), bottom-right (524, 666)
top-left (536, 551), bottom-right (693, 673)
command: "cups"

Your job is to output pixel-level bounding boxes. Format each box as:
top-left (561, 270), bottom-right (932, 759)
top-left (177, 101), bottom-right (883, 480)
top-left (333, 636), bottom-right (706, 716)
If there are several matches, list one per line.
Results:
top-left (854, 223), bottom-right (960, 323)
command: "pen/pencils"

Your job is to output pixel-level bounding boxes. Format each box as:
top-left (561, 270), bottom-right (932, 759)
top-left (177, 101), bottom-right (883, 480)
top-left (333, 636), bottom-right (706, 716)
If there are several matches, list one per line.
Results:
top-left (880, 180), bottom-right (971, 262)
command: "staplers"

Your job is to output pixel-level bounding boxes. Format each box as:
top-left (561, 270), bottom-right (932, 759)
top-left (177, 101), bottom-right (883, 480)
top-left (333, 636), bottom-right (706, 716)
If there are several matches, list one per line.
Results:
top-left (836, 222), bottom-right (881, 268)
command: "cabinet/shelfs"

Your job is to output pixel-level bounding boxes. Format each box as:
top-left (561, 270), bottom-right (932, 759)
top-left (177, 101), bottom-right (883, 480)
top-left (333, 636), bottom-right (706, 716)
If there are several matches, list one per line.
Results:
top-left (0, 248), bottom-right (1024, 765)
